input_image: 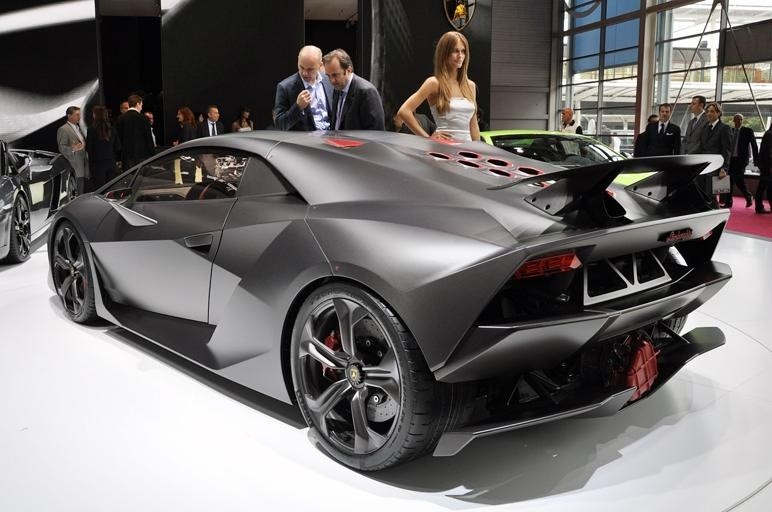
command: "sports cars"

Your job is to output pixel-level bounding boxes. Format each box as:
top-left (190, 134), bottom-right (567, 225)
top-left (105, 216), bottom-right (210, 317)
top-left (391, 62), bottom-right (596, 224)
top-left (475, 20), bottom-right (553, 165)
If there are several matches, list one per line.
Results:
top-left (47, 130), bottom-right (733, 472)
top-left (0, 140), bottom-right (78, 262)
top-left (479, 129), bottom-right (659, 187)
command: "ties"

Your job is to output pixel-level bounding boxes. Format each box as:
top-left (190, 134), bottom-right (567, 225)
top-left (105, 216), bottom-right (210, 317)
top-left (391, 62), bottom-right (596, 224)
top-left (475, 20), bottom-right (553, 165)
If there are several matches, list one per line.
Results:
top-left (211, 123), bottom-right (215, 135)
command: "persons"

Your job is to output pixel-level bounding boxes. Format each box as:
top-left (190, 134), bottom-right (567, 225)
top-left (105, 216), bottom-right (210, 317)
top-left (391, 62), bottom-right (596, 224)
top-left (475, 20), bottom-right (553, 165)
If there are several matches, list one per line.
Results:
top-left (231, 107), bottom-right (254, 133)
top-left (454, 1), bottom-right (467, 28)
top-left (173, 107), bottom-right (201, 147)
top-left (561, 108), bottom-right (583, 135)
top-left (695, 101), bottom-right (731, 198)
top-left (755, 121), bottom-right (772, 213)
top-left (57, 106), bottom-right (89, 197)
top-left (634, 115), bottom-right (659, 157)
top-left (322, 50), bottom-right (386, 131)
top-left (476, 103), bottom-right (489, 131)
top-left (276, 45), bottom-right (332, 133)
top-left (85, 106), bottom-right (115, 193)
top-left (144, 110), bottom-right (165, 156)
top-left (682, 96), bottom-right (710, 155)
top-left (398, 31), bottom-right (482, 141)
top-left (112, 103), bottom-right (129, 129)
top-left (393, 105), bottom-right (437, 137)
top-left (721, 114), bottom-right (758, 208)
top-left (267, 109), bottom-right (277, 129)
top-left (646, 103), bottom-right (682, 156)
top-left (115, 95), bottom-right (155, 173)
top-left (199, 105), bottom-right (225, 138)
